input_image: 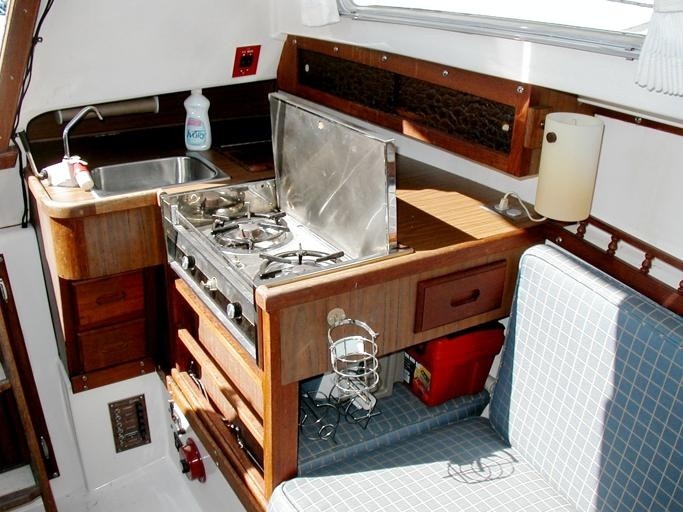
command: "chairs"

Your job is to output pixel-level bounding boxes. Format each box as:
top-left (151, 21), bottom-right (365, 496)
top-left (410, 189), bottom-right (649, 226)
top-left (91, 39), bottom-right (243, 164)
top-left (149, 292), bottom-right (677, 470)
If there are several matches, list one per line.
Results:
top-left (258, 241), bottom-right (683, 512)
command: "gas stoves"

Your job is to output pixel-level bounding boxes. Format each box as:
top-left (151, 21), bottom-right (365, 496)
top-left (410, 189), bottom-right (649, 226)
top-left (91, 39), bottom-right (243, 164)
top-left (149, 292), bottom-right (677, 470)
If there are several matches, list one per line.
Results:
top-left (173, 202), bottom-right (354, 358)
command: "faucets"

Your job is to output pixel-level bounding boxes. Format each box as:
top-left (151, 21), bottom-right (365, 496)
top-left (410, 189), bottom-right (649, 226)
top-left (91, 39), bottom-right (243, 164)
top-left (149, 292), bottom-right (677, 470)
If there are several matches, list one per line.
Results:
top-left (63, 106), bottom-right (104, 158)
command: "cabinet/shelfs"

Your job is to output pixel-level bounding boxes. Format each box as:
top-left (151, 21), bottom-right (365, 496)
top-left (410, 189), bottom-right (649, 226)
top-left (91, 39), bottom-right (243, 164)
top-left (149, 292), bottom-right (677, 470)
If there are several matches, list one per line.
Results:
top-left (58, 264), bottom-right (165, 394)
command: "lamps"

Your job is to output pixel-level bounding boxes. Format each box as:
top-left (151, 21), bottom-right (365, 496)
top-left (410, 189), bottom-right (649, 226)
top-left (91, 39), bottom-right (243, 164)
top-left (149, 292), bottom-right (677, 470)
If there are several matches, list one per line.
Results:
top-left (497, 112), bottom-right (605, 223)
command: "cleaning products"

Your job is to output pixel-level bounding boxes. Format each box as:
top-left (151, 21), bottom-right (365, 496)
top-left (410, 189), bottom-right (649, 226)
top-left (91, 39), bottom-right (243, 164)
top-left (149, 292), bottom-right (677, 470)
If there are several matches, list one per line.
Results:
top-left (183, 88), bottom-right (213, 151)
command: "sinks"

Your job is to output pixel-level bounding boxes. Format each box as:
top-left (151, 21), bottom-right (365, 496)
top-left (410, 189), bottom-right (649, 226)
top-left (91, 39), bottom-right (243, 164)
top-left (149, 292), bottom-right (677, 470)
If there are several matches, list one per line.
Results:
top-left (89, 150), bottom-right (232, 200)
